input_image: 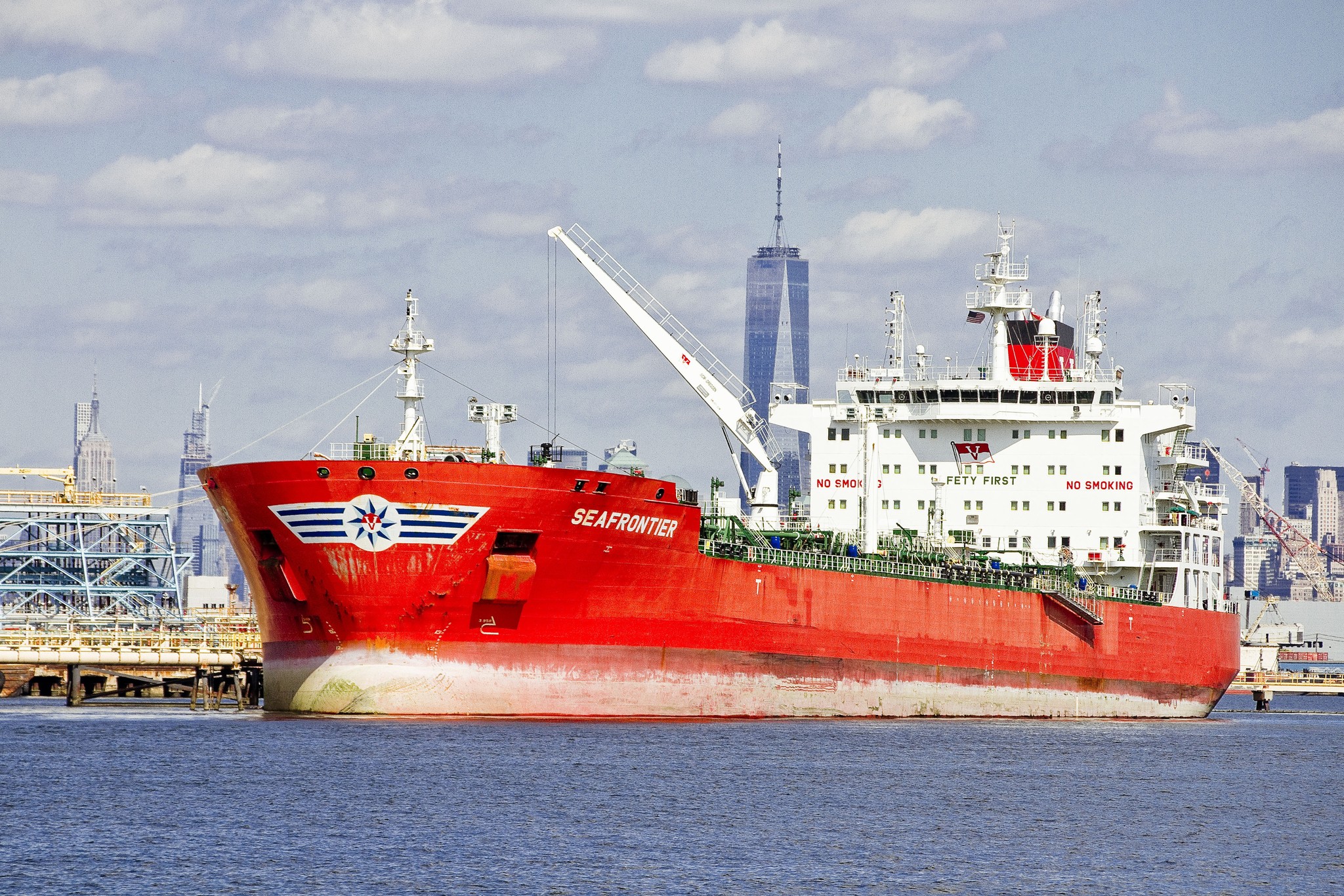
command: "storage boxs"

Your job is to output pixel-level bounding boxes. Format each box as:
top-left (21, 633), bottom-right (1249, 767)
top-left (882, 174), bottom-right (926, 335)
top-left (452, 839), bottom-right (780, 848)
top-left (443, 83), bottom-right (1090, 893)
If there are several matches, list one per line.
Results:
top-left (1246, 671), bottom-right (1265, 683)
top-left (1279, 651), bottom-right (1329, 661)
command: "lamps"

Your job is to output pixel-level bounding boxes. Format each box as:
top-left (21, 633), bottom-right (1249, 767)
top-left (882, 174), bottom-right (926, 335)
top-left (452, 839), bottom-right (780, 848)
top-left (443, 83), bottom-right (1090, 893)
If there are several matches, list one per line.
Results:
top-left (783, 394), bottom-right (792, 402)
top-left (917, 361), bottom-right (924, 368)
top-left (1072, 404), bottom-right (1081, 417)
top-left (1172, 394), bottom-right (1180, 405)
top-left (1182, 396), bottom-right (1190, 405)
top-left (773, 394), bottom-right (781, 403)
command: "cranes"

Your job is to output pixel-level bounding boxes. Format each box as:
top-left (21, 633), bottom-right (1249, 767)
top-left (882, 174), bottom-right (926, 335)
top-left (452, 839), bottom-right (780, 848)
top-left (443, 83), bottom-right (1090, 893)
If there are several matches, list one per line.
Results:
top-left (1234, 436), bottom-right (1271, 543)
top-left (1200, 438), bottom-right (1344, 602)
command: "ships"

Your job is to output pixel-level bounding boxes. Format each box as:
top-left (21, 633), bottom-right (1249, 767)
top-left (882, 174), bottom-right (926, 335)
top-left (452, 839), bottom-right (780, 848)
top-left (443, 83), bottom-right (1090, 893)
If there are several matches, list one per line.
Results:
top-left (196, 209), bottom-right (1239, 721)
